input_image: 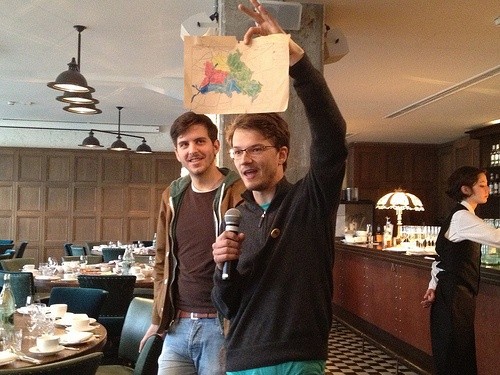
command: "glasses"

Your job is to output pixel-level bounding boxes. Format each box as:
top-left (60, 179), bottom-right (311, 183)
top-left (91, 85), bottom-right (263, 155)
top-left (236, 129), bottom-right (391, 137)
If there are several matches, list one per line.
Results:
top-left (228, 144), bottom-right (280, 159)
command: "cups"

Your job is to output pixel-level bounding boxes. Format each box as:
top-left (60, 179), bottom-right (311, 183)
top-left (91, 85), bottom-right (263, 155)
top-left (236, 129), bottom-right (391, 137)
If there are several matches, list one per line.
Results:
top-left (0, 295), bottom-right (99, 365)
top-left (92, 240), bottom-right (155, 254)
top-left (23, 254), bottom-right (154, 281)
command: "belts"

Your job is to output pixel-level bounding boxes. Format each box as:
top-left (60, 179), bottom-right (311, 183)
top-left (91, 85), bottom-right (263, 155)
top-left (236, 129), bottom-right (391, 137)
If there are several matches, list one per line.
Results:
top-left (176, 310), bottom-right (219, 320)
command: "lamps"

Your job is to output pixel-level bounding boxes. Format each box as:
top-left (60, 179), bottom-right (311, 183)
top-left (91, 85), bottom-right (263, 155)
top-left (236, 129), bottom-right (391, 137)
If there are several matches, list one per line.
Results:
top-left (48, 25), bottom-right (103, 115)
top-left (79, 106), bottom-right (153, 154)
top-left (376, 187), bottom-right (425, 247)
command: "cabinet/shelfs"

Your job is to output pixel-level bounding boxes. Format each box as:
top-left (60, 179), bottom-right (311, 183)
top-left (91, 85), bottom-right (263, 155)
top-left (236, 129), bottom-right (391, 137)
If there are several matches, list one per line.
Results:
top-left (465, 121), bottom-right (500, 219)
top-left (347, 140), bottom-right (480, 209)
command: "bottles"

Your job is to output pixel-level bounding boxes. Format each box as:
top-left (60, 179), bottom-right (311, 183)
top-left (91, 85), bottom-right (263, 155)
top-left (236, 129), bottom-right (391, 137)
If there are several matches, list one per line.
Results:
top-left (479, 141), bottom-right (499, 265)
top-left (365, 216), bottom-right (440, 253)
top-left (0, 272), bottom-right (15, 327)
top-left (152, 233), bottom-right (157, 247)
top-left (124, 248), bottom-right (129, 261)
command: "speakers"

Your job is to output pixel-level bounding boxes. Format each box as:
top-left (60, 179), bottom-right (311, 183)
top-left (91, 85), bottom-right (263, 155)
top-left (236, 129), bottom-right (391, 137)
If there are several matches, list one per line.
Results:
top-left (180, 12), bottom-right (217, 41)
top-left (254, 0), bottom-right (302, 30)
top-left (323, 28), bottom-right (349, 65)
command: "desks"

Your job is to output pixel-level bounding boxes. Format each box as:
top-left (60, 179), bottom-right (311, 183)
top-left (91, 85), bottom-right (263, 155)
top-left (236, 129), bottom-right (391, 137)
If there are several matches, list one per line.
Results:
top-left (0, 308), bottom-right (108, 369)
top-left (91, 249), bottom-right (156, 257)
top-left (33, 263), bottom-right (155, 288)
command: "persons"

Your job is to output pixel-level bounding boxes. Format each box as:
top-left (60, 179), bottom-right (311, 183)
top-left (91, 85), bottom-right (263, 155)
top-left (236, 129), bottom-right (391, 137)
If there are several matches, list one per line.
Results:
top-left (138, 112), bottom-right (246, 375)
top-left (420, 167), bottom-right (500, 375)
top-left (211, 0), bottom-right (349, 375)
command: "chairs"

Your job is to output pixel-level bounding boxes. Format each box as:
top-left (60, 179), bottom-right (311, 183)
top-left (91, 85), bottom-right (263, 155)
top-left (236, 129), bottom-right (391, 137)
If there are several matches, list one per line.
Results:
top-left (48, 288), bottom-right (109, 320)
top-left (0, 271), bottom-right (33, 306)
top-left (96, 297), bottom-right (153, 374)
top-left (134, 336), bottom-right (163, 375)
top-left (0, 351), bottom-right (103, 375)
top-left (16, 240), bottom-right (28, 259)
top-left (0, 249), bottom-right (15, 259)
top-left (0, 239), bottom-right (14, 250)
top-left (0, 258), bottom-right (51, 302)
top-left (76, 274), bottom-right (137, 352)
top-left (61, 240), bottom-right (154, 263)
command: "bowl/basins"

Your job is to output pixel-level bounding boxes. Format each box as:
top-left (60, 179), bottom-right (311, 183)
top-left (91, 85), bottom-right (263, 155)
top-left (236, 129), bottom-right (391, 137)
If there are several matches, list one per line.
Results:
top-left (343, 230), bottom-right (367, 243)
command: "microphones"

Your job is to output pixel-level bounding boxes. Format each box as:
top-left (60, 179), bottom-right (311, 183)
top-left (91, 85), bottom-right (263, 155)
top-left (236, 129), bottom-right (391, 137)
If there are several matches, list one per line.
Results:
top-left (221, 208), bottom-right (243, 280)
